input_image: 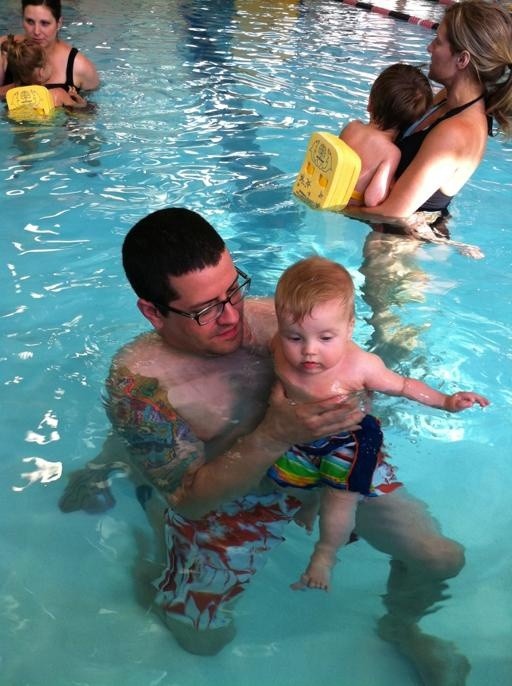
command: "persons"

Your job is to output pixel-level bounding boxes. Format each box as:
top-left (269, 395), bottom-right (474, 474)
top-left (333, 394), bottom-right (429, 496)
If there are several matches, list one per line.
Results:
top-left (243, 255), bottom-right (492, 591)
top-left (100, 206), bottom-right (473, 686)
top-left (359, 0), bottom-right (510, 380)
top-left (2, 36), bottom-right (87, 115)
top-left (2, 0), bottom-right (104, 174)
top-left (336, 63), bottom-right (484, 262)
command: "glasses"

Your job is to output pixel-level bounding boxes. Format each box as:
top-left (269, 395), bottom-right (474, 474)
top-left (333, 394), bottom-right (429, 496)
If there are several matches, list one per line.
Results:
top-left (159, 268), bottom-right (252, 328)
